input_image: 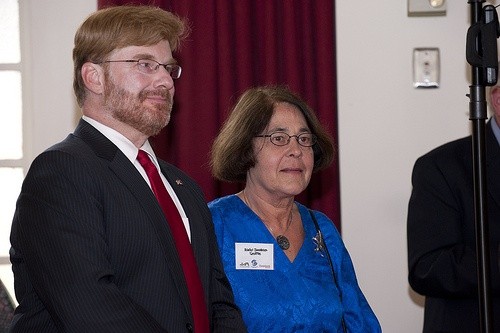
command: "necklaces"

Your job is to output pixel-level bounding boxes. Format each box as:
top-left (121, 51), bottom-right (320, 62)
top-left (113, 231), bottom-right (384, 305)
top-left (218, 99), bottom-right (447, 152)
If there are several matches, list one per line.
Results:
top-left (243, 189), bottom-right (294, 250)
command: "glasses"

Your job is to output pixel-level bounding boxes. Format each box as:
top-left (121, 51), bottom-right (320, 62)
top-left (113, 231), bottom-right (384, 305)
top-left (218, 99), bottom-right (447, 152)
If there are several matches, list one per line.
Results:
top-left (92, 57), bottom-right (183, 80)
top-left (251, 132), bottom-right (317, 147)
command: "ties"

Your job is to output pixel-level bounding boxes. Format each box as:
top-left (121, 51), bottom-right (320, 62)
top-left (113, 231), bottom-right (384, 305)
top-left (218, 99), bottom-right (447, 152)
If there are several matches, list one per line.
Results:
top-left (136, 150), bottom-right (210, 333)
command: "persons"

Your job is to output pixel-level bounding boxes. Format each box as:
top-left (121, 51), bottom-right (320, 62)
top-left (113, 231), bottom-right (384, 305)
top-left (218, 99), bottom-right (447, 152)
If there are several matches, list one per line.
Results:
top-left (406, 64), bottom-right (500, 333)
top-left (10, 6), bottom-right (247, 333)
top-left (206, 84), bottom-right (381, 333)
